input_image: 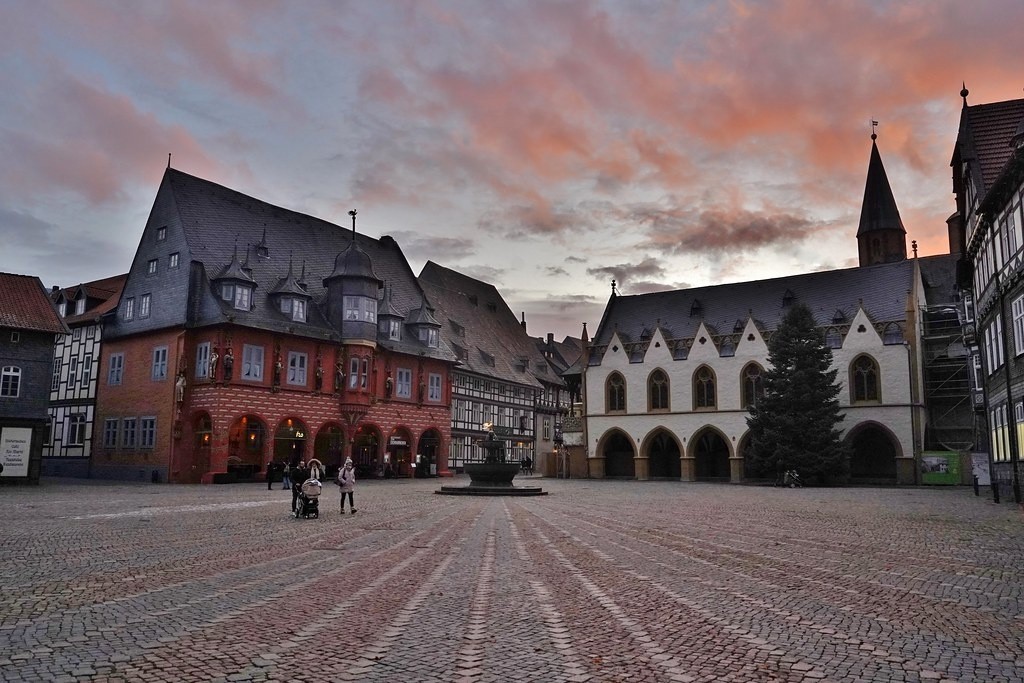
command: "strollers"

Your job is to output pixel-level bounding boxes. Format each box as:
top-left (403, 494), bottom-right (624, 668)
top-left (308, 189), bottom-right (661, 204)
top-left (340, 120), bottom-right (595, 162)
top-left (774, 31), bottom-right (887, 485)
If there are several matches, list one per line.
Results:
top-left (783, 470), bottom-right (804, 488)
top-left (295, 478), bottom-right (322, 518)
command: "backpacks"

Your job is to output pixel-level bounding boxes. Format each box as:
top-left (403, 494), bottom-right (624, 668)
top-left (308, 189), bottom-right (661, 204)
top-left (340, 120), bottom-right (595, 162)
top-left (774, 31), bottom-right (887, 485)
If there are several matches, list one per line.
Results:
top-left (283, 462), bottom-right (291, 473)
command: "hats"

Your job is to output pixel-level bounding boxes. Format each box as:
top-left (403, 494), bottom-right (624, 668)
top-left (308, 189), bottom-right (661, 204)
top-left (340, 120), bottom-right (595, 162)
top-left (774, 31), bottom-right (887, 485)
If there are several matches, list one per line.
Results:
top-left (345, 456), bottom-right (353, 464)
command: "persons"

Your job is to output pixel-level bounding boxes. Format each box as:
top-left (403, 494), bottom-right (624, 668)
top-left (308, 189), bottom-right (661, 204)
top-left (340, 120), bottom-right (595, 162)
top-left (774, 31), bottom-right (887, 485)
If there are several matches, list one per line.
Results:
top-left (307, 460), bottom-right (325, 485)
top-left (222, 347), bottom-right (234, 380)
top-left (265, 460), bottom-right (277, 490)
top-left (175, 369), bottom-right (187, 404)
top-left (280, 457), bottom-right (291, 490)
top-left (521, 457), bottom-right (527, 475)
top-left (525, 455), bottom-right (533, 476)
top-left (336, 456), bottom-right (359, 515)
top-left (418, 374), bottom-right (425, 403)
top-left (289, 460), bottom-right (306, 517)
top-left (315, 360), bottom-right (325, 390)
top-left (207, 347), bottom-right (219, 378)
top-left (385, 369), bottom-right (394, 398)
top-left (334, 359), bottom-right (346, 394)
top-left (271, 355), bottom-right (284, 385)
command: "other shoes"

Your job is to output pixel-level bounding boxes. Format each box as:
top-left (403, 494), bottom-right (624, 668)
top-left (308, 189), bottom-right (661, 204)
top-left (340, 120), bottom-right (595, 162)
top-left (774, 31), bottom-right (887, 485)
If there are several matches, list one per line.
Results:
top-left (350, 506), bottom-right (357, 513)
top-left (340, 508), bottom-right (346, 513)
